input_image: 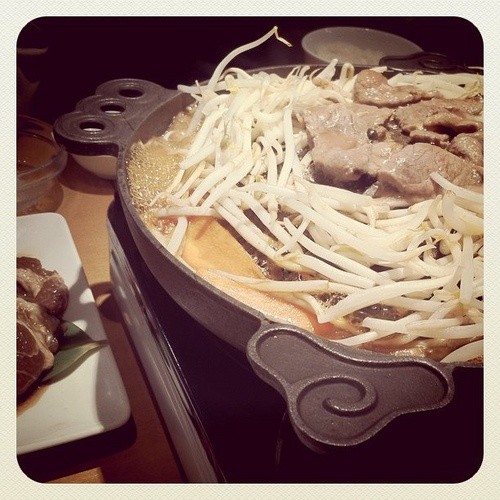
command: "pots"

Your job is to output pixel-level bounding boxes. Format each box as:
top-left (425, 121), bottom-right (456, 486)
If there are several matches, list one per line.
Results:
top-left (50, 52), bottom-right (483, 462)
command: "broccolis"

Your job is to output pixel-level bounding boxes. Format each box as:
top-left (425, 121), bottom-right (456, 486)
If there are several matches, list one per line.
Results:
top-left (37, 319), bottom-right (109, 385)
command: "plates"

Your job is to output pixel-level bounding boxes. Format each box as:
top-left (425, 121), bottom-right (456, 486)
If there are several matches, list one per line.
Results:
top-left (18, 212), bottom-right (132, 454)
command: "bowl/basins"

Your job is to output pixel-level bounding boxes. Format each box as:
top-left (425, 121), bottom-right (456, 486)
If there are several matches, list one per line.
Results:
top-left (301, 27), bottom-right (422, 71)
top-left (17, 108), bottom-right (121, 211)
